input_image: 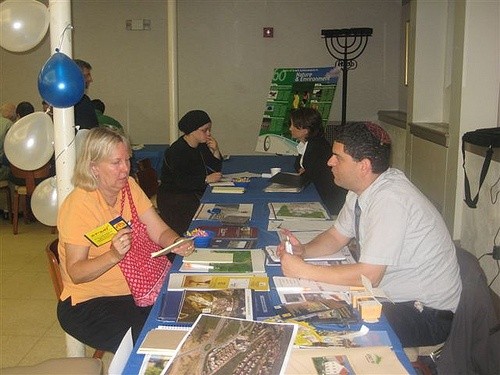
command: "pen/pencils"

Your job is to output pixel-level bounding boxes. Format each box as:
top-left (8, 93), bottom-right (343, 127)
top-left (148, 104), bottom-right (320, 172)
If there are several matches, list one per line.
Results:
top-left (207, 165), bottom-right (226, 178)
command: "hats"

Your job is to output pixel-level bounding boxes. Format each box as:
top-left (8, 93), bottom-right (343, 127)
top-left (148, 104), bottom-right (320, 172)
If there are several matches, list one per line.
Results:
top-left (178, 109), bottom-right (212, 136)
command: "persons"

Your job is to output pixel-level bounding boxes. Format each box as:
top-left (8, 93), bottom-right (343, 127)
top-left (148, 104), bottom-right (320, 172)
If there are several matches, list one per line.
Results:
top-left (276, 120), bottom-right (464, 350)
top-left (292, 88), bottom-right (309, 109)
top-left (156, 110), bottom-right (224, 238)
top-left (56, 123), bottom-right (197, 356)
top-left (0, 98), bottom-right (124, 221)
top-left (288, 107), bottom-right (348, 214)
top-left (206, 207), bottom-right (225, 215)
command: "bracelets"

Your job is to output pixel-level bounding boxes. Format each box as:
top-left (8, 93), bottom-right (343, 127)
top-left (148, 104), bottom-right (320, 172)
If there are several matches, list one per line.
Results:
top-left (205, 177), bottom-right (208, 184)
top-left (171, 237), bottom-right (183, 243)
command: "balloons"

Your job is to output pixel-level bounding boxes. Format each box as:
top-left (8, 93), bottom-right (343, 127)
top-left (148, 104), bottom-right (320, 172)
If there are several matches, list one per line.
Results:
top-left (0, 0), bottom-right (50, 52)
top-left (37, 48), bottom-right (86, 108)
top-left (3, 111), bottom-right (56, 171)
top-left (76, 125), bottom-right (90, 162)
top-left (30, 177), bottom-right (58, 226)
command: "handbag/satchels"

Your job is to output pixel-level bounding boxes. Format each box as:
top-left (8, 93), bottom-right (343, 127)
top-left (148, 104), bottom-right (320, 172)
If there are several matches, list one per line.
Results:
top-left (462, 127), bottom-right (500, 148)
top-left (110, 176), bottom-right (173, 308)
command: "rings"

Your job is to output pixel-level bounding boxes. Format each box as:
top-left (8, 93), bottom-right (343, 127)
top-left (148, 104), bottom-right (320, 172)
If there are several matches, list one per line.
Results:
top-left (119, 237), bottom-right (124, 243)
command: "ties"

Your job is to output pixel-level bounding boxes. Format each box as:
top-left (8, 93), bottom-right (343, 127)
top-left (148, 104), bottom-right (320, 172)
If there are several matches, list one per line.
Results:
top-left (354, 199), bottom-right (362, 246)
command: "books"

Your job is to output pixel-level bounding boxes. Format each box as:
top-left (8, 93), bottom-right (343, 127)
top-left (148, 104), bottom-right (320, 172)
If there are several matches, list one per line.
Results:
top-left (209, 166), bottom-right (305, 195)
top-left (136, 202), bottom-right (410, 375)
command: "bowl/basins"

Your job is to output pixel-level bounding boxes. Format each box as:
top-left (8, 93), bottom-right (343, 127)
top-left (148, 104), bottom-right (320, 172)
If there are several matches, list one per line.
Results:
top-left (233, 178), bottom-right (250, 187)
top-left (184, 230), bottom-right (216, 247)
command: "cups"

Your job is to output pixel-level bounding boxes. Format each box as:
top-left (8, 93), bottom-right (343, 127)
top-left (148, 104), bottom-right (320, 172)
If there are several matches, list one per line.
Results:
top-left (271, 168), bottom-right (281, 176)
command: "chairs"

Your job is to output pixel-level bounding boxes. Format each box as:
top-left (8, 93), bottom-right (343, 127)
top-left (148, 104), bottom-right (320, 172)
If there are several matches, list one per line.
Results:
top-left (45, 238), bottom-right (107, 358)
top-left (8, 160), bottom-right (55, 235)
top-left (134, 169), bottom-right (159, 209)
top-left (404, 244), bottom-right (500, 375)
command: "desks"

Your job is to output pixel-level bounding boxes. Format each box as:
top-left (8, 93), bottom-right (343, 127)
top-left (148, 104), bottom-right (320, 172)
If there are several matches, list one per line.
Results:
top-left (124, 155), bottom-right (416, 375)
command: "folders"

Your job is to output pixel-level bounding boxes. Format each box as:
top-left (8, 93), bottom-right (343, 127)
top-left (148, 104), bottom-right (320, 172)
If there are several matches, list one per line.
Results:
top-left (261, 172), bottom-right (304, 194)
